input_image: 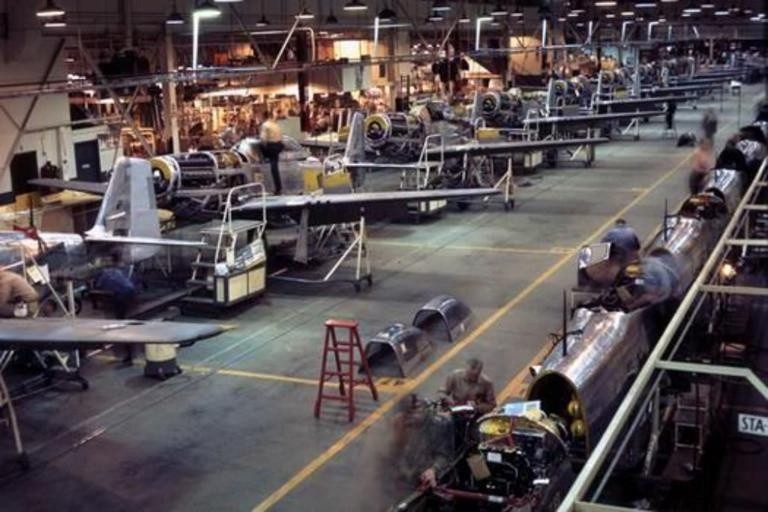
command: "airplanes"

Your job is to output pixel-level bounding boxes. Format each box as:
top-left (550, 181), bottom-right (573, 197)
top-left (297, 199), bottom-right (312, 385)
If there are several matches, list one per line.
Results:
top-left (33, 108), bottom-right (502, 296)
top-left (0, 155), bottom-right (239, 474)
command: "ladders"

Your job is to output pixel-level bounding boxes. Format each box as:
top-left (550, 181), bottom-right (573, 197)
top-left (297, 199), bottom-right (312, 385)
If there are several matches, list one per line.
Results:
top-left (313, 320), bottom-right (378, 423)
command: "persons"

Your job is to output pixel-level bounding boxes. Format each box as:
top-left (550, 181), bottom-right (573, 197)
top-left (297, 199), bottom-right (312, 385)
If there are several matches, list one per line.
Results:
top-left (430, 46), bottom-right (458, 102)
top-left (661, 93), bottom-right (677, 129)
top-left (436, 358), bottom-right (496, 446)
top-left (702, 106), bottom-right (719, 142)
top-left (621, 255), bottom-right (684, 323)
top-left (246, 119), bottom-right (288, 198)
top-left (1, 265), bottom-right (43, 317)
top-left (599, 218), bottom-right (641, 265)
top-left (686, 135), bottom-right (718, 193)
top-left (94, 253), bottom-right (139, 372)
top-left (385, 393), bottom-right (457, 493)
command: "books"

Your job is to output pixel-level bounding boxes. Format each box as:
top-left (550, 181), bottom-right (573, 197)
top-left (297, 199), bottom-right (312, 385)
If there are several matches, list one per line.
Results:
top-left (447, 403), bottom-right (476, 415)
